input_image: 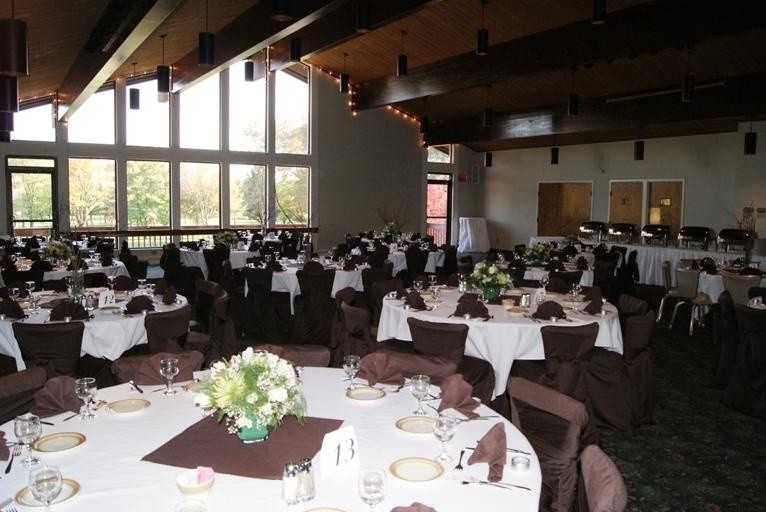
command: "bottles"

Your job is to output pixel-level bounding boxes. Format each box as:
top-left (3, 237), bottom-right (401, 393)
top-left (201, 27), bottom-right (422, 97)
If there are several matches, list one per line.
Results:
top-left (281, 455), bottom-right (315, 505)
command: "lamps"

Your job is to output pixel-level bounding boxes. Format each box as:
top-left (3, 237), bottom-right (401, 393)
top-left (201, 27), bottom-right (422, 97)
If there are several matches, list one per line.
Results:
top-left (419, 97), bottom-right (430, 134)
top-left (680, 47), bottom-right (696, 103)
top-left (395, 30), bottom-right (409, 77)
top-left (484, 144), bottom-right (494, 169)
top-left (566, 70), bottom-right (580, 117)
top-left (591, 1), bottom-right (608, 26)
top-left (198, 1), bottom-right (215, 68)
top-left (130, 61), bottom-right (140, 110)
top-left (633, 137), bottom-right (645, 161)
top-left (0, 113), bottom-right (14, 132)
top-left (0, 75), bottom-right (21, 113)
top-left (549, 138), bottom-right (560, 164)
top-left (481, 85), bottom-right (495, 128)
top-left (339, 51), bottom-right (349, 94)
top-left (475, 2), bottom-right (489, 57)
top-left (0, 1), bottom-right (31, 78)
top-left (0, 131), bottom-right (12, 144)
top-left (742, 121), bottom-right (758, 156)
top-left (288, 33), bottom-right (302, 63)
top-left (244, 57), bottom-right (255, 81)
top-left (355, 1), bottom-right (373, 34)
top-left (157, 33), bottom-right (170, 94)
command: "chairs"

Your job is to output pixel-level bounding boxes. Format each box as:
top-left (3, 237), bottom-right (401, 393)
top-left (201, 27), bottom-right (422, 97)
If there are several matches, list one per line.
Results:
top-left (0, 215), bottom-right (765, 511)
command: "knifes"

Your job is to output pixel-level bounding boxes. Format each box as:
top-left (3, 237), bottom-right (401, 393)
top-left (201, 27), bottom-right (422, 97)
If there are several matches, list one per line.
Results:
top-left (152, 385), bottom-right (176, 392)
top-left (455, 415), bottom-right (499, 424)
top-left (129, 380), bottom-right (143, 393)
top-left (17, 418), bottom-right (54, 425)
top-left (478, 480), bottom-right (511, 489)
top-left (476, 439), bottom-right (526, 453)
top-left (63, 412), bottom-right (79, 421)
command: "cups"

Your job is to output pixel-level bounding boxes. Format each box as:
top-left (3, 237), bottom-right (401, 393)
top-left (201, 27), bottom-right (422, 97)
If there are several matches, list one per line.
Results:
top-left (195, 240), bottom-right (209, 249)
top-left (511, 455), bottom-right (530, 471)
top-left (177, 470), bottom-right (214, 502)
top-left (173, 498), bottom-right (208, 512)
top-left (237, 229), bottom-right (290, 240)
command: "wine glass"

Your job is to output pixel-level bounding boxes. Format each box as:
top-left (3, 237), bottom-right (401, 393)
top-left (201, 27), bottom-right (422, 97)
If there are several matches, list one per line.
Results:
top-left (13, 415), bottom-right (41, 465)
top-left (6, 275), bottom-right (156, 322)
top-left (494, 249), bottom-right (576, 270)
top-left (2, 234), bottom-right (103, 269)
top-left (705, 255), bottom-right (733, 271)
top-left (365, 231), bottom-right (430, 253)
top-left (74, 377), bottom-right (98, 421)
top-left (391, 271), bottom-right (585, 322)
top-left (433, 414), bottom-right (457, 461)
top-left (159, 359), bottom-right (180, 397)
top-left (357, 467), bottom-right (387, 512)
top-left (343, 354), bottom-right (362, 391)
top-left (263, 249), bottom-right (352, 269)
top-left (410, 374), bottom-right (431, 415)
top-left (29, 465), bottom-right (62, 512)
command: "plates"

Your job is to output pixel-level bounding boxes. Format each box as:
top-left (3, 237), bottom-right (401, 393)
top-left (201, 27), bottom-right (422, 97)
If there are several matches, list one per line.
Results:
top-left (106, 399), bottom-right (151, 414)
top-left (388, 457), bottom-right (443, 482)
top-left (395, 415), bottom-right (440, 435)
top-left (346, 386), bottom-right (386, 401)
top-left (30, 431), bottom-right (86, 454)
top-left (16, 479), bottom-right (80, 507)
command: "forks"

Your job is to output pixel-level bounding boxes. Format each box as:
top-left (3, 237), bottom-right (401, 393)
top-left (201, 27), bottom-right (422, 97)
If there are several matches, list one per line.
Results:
top-left (580, 311), bottom-right (602, 318)
top-left (4, 445), bottom-right (22, 474)
top-left (453, 449), bottom-right (464, 470)
